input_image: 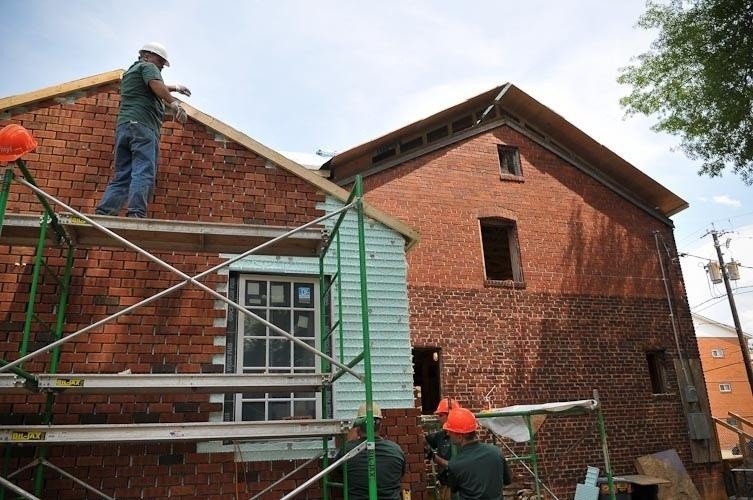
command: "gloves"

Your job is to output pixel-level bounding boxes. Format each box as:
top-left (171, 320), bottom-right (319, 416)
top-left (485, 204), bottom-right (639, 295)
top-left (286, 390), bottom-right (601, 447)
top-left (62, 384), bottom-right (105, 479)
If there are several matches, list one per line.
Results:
top-left (170, 101), bottom-right (187, 124)
top-left (175, 84), bottom-right (191, 97)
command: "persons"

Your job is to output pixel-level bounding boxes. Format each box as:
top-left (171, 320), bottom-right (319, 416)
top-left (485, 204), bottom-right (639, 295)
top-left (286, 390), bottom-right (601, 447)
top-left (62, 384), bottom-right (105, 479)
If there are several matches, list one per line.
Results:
top-left (94, 43), bottom-right (190, 219)
top-left (330, 402), bottom-right (406, 500)
top-left (423, 397), bottom-right (460, 500)
top-left (422, 407), bottom-right (513, 500)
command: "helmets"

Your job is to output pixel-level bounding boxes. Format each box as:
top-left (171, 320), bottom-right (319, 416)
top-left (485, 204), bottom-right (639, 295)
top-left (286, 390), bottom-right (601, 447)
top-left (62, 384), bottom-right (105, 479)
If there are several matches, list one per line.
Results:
top-left (358, 404), bottom-right (384, 418)
top-left (0, 124), bottom-right (37, 161)
top-left (139, 41), bottom-right (170, 67)
top-left (434, 398), bottom-right (478, 435)
